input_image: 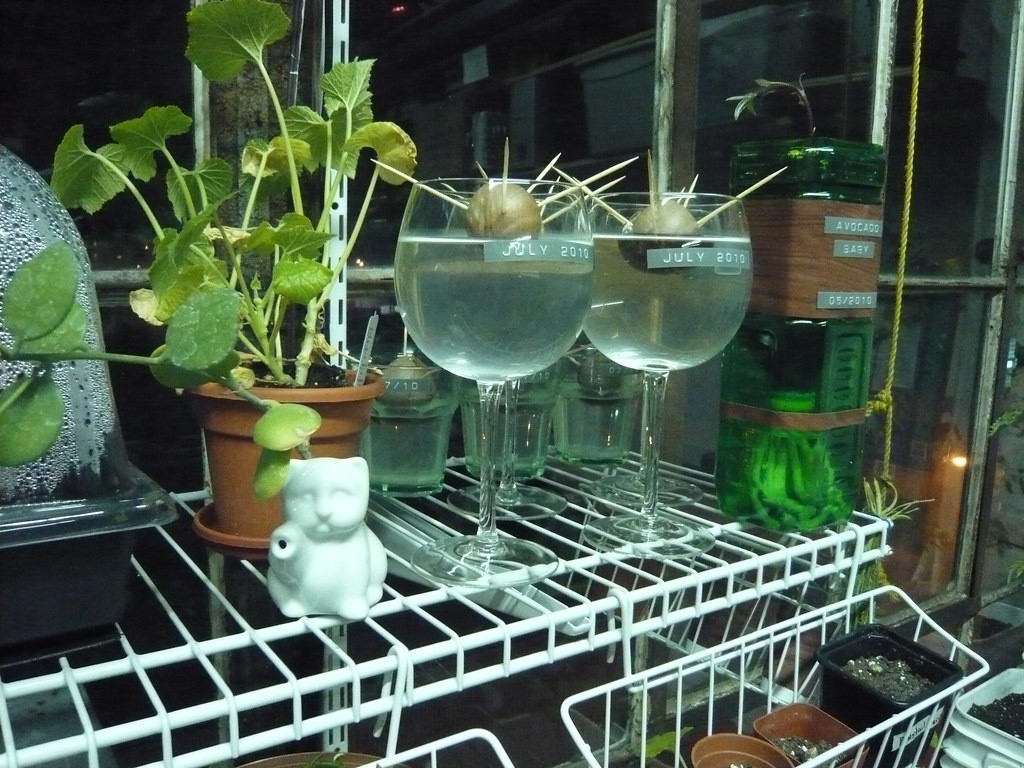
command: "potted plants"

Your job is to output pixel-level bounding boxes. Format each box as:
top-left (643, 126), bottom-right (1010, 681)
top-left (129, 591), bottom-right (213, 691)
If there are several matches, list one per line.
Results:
top-left (753, 702), bottom-right (871, 768)
top-left (942, 668), bottom-right (1024, 768)
top-left (0, 0), bottom-right (423, 564)
top-left (726, 74), bottom-right (890, 534)
top-left (815, 623), bottom-right (965, 768)
top-left (691, 733), bottom-right (796, 768)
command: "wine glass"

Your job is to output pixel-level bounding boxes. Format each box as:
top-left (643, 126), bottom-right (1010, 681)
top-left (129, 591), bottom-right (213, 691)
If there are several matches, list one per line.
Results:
top-left (585, 193), bottom-right (752, 561)
top-left (393, 175), bottom-right (595, 590)
top-left (593, 204), bottom-right (723, 510)
top-left (441, 196), bottom-right (571, 524)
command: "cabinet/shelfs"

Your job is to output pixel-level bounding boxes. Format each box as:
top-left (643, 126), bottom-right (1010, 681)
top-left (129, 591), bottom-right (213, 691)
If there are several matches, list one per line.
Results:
top-left (0, 438), bottom-right (990, 768)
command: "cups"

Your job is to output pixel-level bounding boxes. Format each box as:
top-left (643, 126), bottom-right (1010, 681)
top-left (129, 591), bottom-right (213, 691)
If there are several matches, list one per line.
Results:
top-left (556, 347), bottom-right (645, 463)
top-left (345, 363), bottom-right (459, 492)
top-left (453, 358), bottom-right (564, 482)
